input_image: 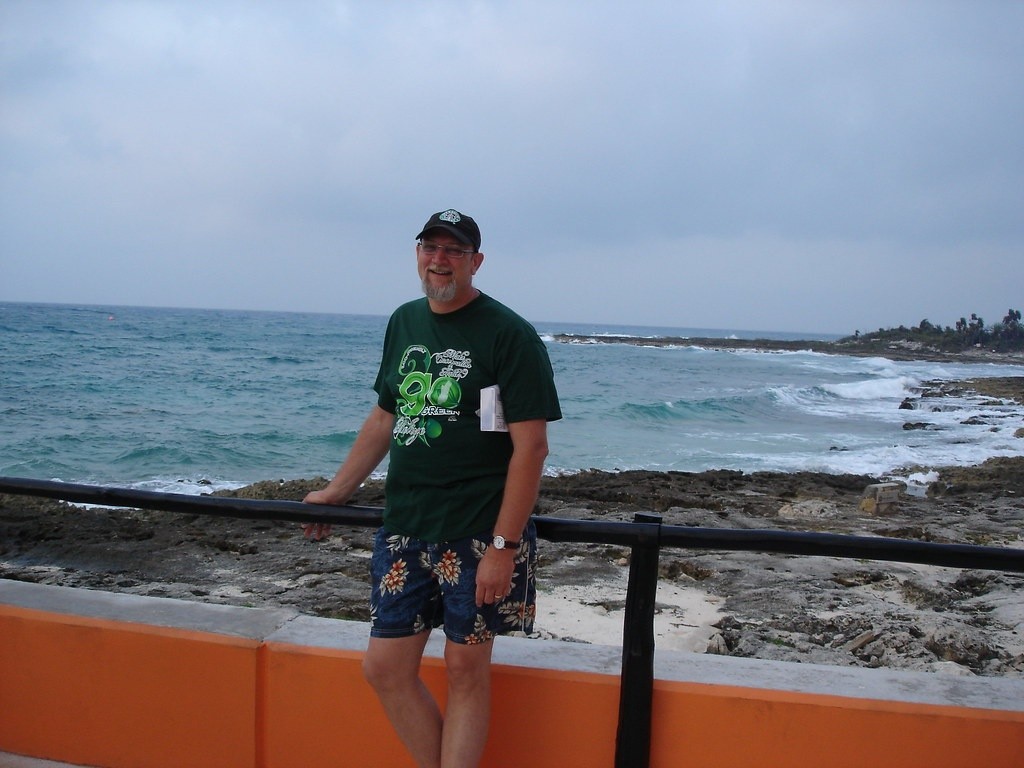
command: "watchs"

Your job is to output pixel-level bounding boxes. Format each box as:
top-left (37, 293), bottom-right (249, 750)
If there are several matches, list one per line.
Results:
top-left (491, 535), bottom-right (520, 550)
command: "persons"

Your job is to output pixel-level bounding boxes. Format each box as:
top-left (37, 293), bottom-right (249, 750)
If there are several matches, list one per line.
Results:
top-left (302, 209), bottom-right (562, 768)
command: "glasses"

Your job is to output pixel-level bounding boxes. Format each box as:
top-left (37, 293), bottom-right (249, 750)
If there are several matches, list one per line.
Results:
top-left (417, 242), bottom-right (478, 258)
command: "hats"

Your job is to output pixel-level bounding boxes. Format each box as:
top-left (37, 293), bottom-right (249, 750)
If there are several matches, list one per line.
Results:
top-left (415, 208), bottom-right (481, 248)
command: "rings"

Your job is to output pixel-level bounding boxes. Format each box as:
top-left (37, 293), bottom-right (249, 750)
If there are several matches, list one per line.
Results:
top-left (495, 595), bottom-right (502, 598)
